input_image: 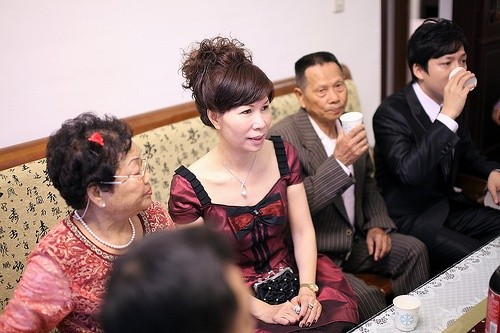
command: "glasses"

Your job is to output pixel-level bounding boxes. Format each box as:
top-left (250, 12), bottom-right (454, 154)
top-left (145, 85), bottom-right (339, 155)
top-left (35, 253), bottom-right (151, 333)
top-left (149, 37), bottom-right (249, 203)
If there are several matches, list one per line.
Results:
top-left (100, 158), bottom-right (148, 185)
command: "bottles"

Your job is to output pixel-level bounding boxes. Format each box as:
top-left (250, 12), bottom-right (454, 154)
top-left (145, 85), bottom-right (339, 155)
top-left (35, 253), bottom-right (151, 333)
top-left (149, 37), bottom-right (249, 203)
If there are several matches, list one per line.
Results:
top-left (484, 264), bottom-right (500, 333)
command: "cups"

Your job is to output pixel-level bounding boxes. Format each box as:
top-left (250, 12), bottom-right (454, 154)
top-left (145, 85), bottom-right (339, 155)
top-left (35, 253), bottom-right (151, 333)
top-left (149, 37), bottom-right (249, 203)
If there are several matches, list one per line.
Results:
top-left (448, 66), bottom-right (477, 91)
top-left (339, 112), bottom-right (364, 137)
top-left (393, 295), bottom-right (422, 332)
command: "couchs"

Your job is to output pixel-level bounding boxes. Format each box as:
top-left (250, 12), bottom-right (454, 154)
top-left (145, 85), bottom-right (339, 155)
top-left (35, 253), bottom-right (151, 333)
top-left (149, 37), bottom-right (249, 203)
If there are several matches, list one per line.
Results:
top-left (0, 64), bottom-right (373, 333)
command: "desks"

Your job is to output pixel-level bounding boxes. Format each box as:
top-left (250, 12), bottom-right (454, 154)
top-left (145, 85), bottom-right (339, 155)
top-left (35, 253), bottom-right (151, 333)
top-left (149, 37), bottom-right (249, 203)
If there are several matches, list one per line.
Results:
top-left (349, 234), bottom-right (500, 333)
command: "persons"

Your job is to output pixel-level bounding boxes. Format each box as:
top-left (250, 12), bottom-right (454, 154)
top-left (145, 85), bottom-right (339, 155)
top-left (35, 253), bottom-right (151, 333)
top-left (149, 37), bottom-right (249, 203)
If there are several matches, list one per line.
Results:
top-left (373, 17), bottom-right (500, 260)
top-left (168, 33), bottom-right (360, 333)
top-left (266, 51), bottom-right (431, 319)
top-left (0, 110), bottom-right (176, 333)
top-left (100, 226), bottom-right (253, 333)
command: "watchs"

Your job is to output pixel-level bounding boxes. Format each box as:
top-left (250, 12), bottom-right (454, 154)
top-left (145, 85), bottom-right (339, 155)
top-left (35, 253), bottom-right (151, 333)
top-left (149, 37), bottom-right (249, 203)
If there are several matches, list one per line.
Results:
top-left (298, 282), bottom-right (319, 293)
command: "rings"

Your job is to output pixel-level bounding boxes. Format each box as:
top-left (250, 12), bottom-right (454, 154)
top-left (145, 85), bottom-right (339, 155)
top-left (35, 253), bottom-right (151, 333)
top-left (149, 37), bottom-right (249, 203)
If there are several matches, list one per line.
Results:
top-left (293, 304), bottom-right (302, 312)
top-left (308, 301), bottom-right (315, 310)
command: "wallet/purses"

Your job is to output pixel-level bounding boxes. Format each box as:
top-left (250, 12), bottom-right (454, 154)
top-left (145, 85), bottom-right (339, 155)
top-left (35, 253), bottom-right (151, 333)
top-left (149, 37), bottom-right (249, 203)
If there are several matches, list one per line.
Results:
top-left (253, 267), bottom-right (300, 305)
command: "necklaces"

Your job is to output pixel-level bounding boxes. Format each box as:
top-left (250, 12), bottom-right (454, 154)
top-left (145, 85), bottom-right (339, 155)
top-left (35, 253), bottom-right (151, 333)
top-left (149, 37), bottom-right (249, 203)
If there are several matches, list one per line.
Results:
top-left (218, 146), bottom-right (258, 196)
top-left (75, 209), bottom-right (136, 249)
